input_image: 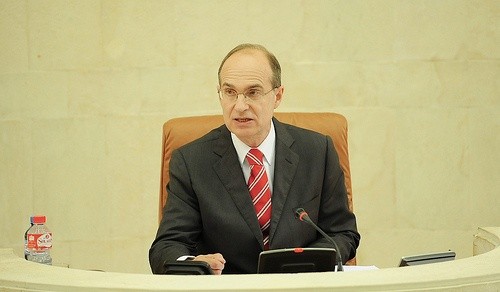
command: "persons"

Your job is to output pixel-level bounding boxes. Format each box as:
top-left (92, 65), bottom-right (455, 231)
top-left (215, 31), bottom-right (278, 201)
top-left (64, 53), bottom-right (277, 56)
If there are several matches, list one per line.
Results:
top-left (149, 44), bottom-right (362, 274)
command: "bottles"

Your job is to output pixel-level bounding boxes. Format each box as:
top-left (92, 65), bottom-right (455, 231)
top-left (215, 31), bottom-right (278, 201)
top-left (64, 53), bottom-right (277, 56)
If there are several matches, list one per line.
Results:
top-left (27, 215), bottom-right (52, 265)
top-left (24, 216), bottom-right (33, 259)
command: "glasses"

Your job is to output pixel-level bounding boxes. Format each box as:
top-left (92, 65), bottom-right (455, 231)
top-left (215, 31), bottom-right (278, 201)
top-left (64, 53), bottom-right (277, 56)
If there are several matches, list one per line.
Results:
top-left (217, 86), bottom-right (279, 104)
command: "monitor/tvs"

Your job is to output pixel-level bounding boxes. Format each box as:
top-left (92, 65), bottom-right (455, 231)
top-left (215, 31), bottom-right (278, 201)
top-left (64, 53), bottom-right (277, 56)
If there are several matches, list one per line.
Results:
top-left (256, 247), bottom-right (336, 273)
top-left (399, 251), bottom-right (456, 266)
top-left (163, 260), bottom-right (215, 275)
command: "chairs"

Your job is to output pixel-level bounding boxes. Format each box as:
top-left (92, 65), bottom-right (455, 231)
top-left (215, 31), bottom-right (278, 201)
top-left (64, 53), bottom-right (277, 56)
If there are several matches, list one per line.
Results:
top-left (161, 112), bottom-right (357, 267)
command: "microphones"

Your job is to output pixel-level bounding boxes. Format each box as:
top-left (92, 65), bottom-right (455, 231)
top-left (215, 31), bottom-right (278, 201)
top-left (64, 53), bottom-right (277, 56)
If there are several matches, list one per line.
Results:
top-left (294, 207), bottom-right (344, 271)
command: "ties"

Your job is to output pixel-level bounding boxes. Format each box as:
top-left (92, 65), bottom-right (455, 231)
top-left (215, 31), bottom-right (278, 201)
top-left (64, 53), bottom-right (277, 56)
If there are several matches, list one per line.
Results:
top-left (245, 149), bottom-right (272, 251)
